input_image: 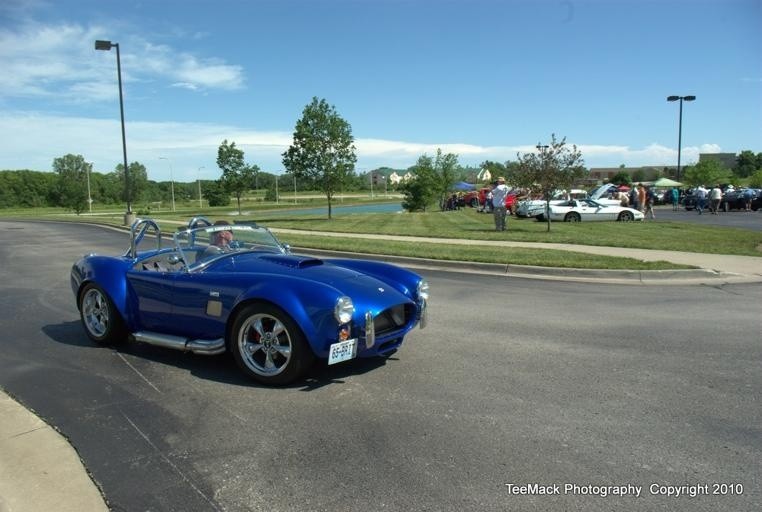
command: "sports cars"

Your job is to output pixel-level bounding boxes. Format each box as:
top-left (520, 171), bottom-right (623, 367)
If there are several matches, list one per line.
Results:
top-left (71, 216), bottom-right (430, 388)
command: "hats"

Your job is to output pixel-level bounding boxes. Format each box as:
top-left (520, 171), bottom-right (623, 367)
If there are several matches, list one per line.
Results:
top-left (497, 177), bottom-right (506, 182)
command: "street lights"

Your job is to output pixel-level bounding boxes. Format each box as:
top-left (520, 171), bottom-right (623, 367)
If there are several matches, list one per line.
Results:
top-left (159, 157), bottom-right (175, 211)
top-left (87, 161), bottom-right (95, 212)
top-left (199, 166), bottom-right (205, 208)
top-left (95, 40), bottom-right (133, 214)
top-left (667, 96), bottom-right (695, 190)
top-left (536, 146), bottom-right (549, 170)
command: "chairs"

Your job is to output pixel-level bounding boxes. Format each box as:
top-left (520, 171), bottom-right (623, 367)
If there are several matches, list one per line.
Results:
top-left (134, 247), bottom-right (204, 272)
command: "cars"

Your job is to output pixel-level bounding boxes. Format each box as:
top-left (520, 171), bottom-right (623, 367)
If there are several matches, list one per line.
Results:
top-left (654, 194), bottom-right (666, 205)
top-left (505, 183), bottom-right (645, 222)
top-left (682, 189), bottom-right (762, 211)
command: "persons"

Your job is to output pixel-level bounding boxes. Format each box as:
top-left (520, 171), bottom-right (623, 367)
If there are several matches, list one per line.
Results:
top-left (445, 178), bottom-right (756, 232)
top-left (201, 220), bottom-right (238, 262)
